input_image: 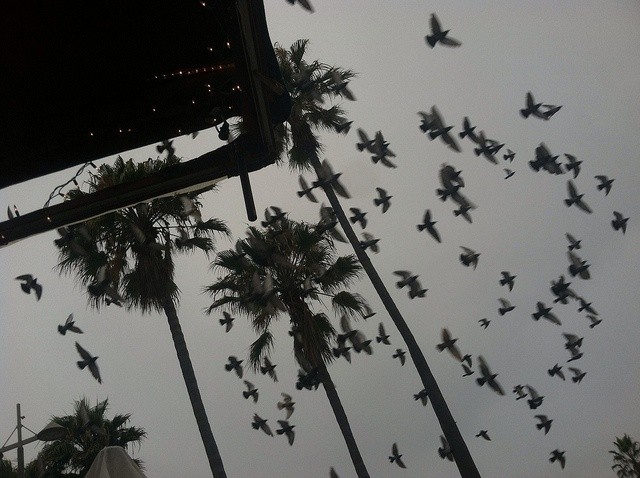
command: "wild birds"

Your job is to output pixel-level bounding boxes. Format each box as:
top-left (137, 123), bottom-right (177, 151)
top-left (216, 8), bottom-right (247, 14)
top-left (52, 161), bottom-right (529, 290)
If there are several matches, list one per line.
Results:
top-left (531, 301), bottom-right (561, 325)
top-left (547, 363), bottom-right (566, 381)
top-left (356, 127), bottom-right (398, 169)
top-left (499, 272), bottom-right (518, 293)
top-left (459, 246), bottom-right (481, 270)
top-left (156, 138), bottom-right (175, 160)
top-left (594, 175), bottom-right (616, 197)
top-left (512, 383), bottom-right (545, 410)
top-left (503, 148), bottom-right (516, 163)
top-left (413, 387), bottom-right (429, 407)
top-left (475, 429), bottom-right (492, 441)
top-left (332, 314), bottom-right (374, 365)
top-left (461, 353), bottom-right (473, 369)
top-left (539, 104), bottom-right (565, 120)
top-left (312, 158), bottom-right (350, 199)
top-left (458, 115), bottom-right (480, 144)
top-left (563, 152), bottom-right (584, 180)
top-left (578, 297), bottom-right (599, 315)
top-left (224, 356), bottom-right (245, 379)
top-left (476, 355), bottom-right (506, 396)
top-left (567, 367), bottom-right (588, 384)
top-left (416, 208), bottom-right (442, 243)
top-left (611, 210), bottom-right (631, 235)
top-left (585, 315), bottom-right (603, 329)
top-left (502, 168), bottom-right (516, 179)
top-left (527, 140), bottom-right (567, 176)
top-left (568, 252), bottom-right (592, 281)
top-left (359, 231), bottom-right (382, 254)
top-left (275, 420), bottom-right (296, 447)
top-left (259, 355), bottom-right (280, 383)
top-left (461, 364), bottom-right (476, 378)
top-left (277, 392), bottom-right (296, 420)
top-left (375, 322), bottom-right (392, 345)
top-left (436, 164), bottom-right (478, 225)
top-left (478, 318), bottom-right (491, 330)
top-left (349, 207), bottom-right (368, 230)
top-left (271, 206), bottom-right (288, 224)
top-left (242, 380), bottom-right (259, 404)
top-left (392, 348), bottom-right (407, 367)
top-left (533, 415), bottom-right (555, 436)
top-left (437, 328), bottom-right (464, 363)
top-left (296, 174), bottom-right (320, 203)
top-left (497, 297), bottom-right (517, 316)
top-left (57, 312), bottom-right (85, 336)
top-left (551, 276), bottom-right (579, 305)
top-left (548, 448), bottom-right (567, 470)
top-left (424, 13), bottom-right (464, 48)
top-left (562, 333), bottom-right (585, 363)
top-left (261, 207), bottom-right (282, 230)
top-left (565, 231), bottom-right (584, 252)
top-left (392, 270), bottom-right (430, 300)
top-left (373, 186), bottom-right (395, 214)
top-left (474, 129), bottom-right (506, 166)
top-left (286, 0), bottom-right (314, 13)
top-left (438, 434), bottom-right (454, 462)
top-left (14, 274), bottom-right (43, 301)
top-left (388, 442), bottom-right (408, 469)
top-left (219, 311), bottom-right (236, 333)
top-left (519, 91), bottom-right (550, 120)
top-left (563, 179), bottom-right (594, 214)
top-left (416, 104), bottom-right (464, 154)
top-left (74, 341), bottom-right (102, 385)
top-left (251, 412), bottom-right (274, 438)
